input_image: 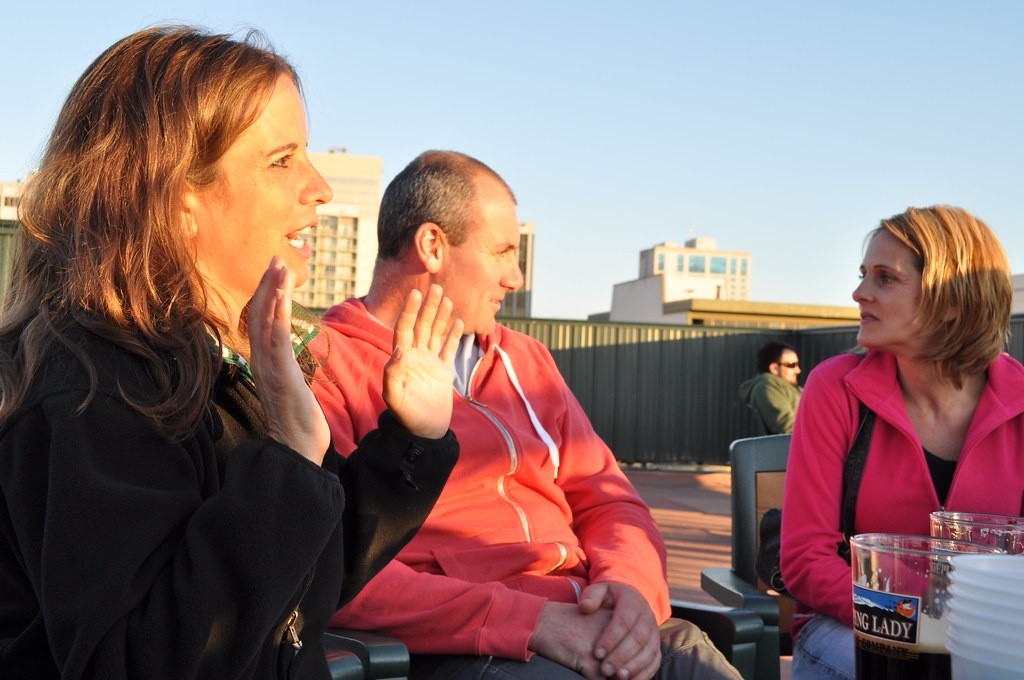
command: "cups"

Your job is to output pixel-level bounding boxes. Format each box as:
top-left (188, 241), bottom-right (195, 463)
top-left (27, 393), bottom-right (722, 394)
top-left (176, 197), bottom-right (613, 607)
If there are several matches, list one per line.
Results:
top-left (927, 511), bottom-right (1024, 627)
top-left (849, 532), bottom-right (1009, 680)
top-left (942, 553), bottom-right (1024, 680)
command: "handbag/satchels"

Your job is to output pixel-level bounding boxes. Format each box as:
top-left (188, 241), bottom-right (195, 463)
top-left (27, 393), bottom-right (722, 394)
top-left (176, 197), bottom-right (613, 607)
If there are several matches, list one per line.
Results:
top-left (753, 349), bottom-right (876, 608)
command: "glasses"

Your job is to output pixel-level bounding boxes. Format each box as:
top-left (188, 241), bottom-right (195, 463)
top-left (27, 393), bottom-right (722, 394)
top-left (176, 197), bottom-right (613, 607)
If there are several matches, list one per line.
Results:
top-left (775, 360), bottom-right (801, 368)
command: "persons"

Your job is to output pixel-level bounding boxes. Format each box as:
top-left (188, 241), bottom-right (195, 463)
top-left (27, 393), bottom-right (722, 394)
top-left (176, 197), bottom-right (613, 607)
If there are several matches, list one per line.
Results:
top-left (307, 148), bottom-right (744, 680)
top-left (739, 340), bottom-right (804, 434)
top-left (779, 204), bottom-right (1024, 680)
top-left (0, 17), bottom-right (464, 680)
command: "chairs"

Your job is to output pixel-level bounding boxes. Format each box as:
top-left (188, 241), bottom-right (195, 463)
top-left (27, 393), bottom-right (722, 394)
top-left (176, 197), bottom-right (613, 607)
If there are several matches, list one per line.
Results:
top-left (745, 403), bottom-right (767, 436)
top-left (327, 599), bottom-right (763, 680)
top-left (699, 433), bottom-right (792, 680)
